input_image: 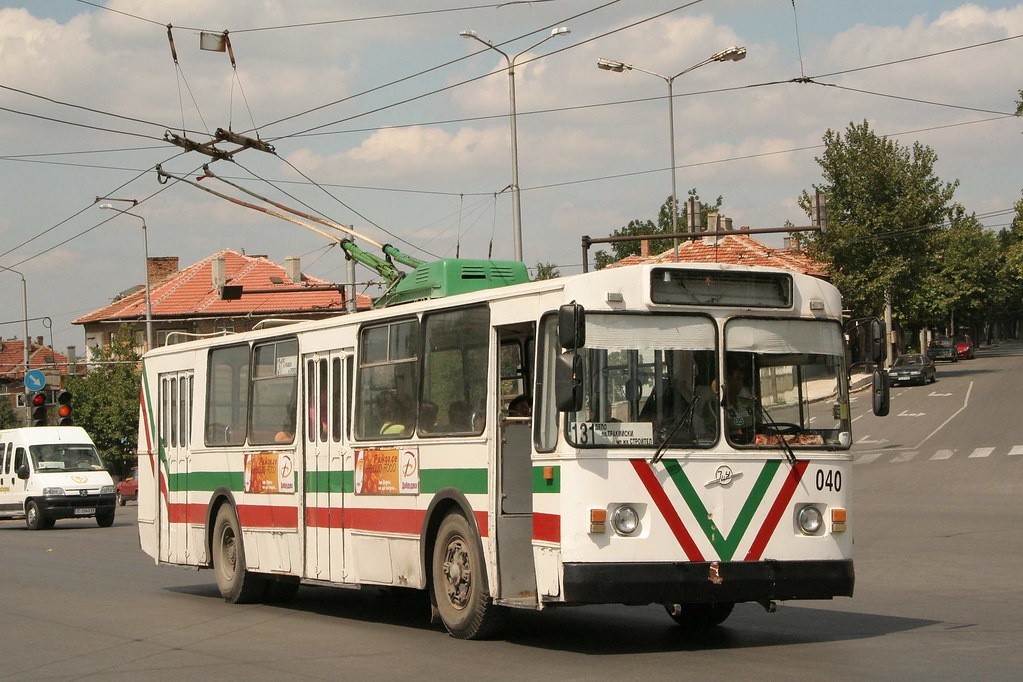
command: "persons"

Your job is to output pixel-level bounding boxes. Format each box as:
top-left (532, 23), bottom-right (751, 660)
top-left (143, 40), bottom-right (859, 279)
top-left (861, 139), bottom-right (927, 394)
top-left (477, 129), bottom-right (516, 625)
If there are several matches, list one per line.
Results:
top-left (964, 333), bottom-right (970, 342)
top-left (447, 399), bottom-right (471, 423)
top-left (586, 400), bottom-right (621, 424)
top-left (307, 372), bottom-right (327, 442)
top-left (507, 398), bottom-right (530, 417)
top-left (364, 391), bottom-right (439, 435)
top-left (704, 363), bottom-right (774, 441)
top-left (273, 405), bottom-right (304, 444)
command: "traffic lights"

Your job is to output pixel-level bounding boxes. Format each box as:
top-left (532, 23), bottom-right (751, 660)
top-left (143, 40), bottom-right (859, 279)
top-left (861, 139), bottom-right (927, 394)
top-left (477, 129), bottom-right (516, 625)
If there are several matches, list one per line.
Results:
top-left (30, 392), bottom-right (47, 427)
top-left (56, 391), bottom-right (73, 425)
top-left (220, 285), bottom-right (243, 300)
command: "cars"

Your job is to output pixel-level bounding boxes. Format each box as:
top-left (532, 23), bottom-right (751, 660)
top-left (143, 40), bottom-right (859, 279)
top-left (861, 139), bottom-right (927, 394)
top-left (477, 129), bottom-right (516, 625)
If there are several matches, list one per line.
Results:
top-left (927, 338), bottom-right (958, 362)
top-left (949, 334), bottom-right (975, 360)
top-left (116, 467), bottom-right (138, 506)
top-left (888, 354), bottom-right (936, 387)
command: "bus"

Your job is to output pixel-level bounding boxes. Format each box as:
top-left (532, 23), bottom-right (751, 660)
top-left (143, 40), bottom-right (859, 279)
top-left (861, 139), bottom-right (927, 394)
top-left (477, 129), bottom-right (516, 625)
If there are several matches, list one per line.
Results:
top-left (137, 239), bottom-right (890, 640)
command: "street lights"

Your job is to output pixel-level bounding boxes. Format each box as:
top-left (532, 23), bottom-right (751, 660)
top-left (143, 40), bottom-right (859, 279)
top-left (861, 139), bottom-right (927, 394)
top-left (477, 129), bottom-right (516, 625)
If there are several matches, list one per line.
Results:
top-left (460, 27), bottom-right (571, 263)
top-left (596, 47), bottom-right (746, 263)
top-left (99, 204), bottom-right (154, 351)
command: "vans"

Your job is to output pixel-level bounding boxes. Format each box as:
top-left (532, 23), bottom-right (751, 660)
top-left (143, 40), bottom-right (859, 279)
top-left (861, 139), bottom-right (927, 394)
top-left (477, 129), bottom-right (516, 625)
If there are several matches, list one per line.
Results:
top-left (0, 426), bottom-right (116, 530)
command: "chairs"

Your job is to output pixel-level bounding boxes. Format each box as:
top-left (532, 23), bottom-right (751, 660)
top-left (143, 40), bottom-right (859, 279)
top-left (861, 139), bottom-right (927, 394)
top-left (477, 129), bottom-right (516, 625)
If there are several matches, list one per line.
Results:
top-left (916, 358), bottom-right (921, 363)
top-left (205, 401), bottom-right (525, 444)
